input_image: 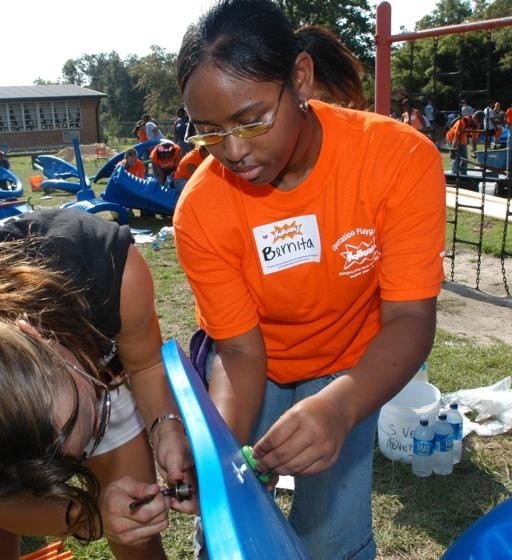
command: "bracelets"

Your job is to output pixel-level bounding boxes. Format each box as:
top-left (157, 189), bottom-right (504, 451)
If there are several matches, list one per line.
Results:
top-left (66, 494), bottom-right (86, 540)
top-left (147, 414), bottom-right (184, 450)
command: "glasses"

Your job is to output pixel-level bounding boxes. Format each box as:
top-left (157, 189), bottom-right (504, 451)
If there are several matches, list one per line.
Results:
top-left (70, 363), bottom-right (111, 464)
top-left (184, 84), bottom-right (286, 146)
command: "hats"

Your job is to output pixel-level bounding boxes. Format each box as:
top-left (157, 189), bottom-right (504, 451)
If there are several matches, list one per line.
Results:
top-left (476, 110), bottom-right (485, 126)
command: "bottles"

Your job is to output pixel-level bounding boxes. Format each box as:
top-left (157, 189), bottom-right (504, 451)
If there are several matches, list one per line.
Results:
top-left (412, 402), bottom-right (463, 477)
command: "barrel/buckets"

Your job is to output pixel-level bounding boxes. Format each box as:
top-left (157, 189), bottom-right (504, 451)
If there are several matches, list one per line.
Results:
top-left (29, 175), bottom-right (44, 192)
top-left (376, 380), bottom-right (442, 466)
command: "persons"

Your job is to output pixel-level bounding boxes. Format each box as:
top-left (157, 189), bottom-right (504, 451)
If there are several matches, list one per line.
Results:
top-left (0, 151), bottom-right (9, 169)
top-left (390, 99), bottom-right (512, 175)
top-left (0, 208), bottom-right (200, 560)
top-left (115, 107), bottom-right (196, 218)
top-left (172, 0), bottom-right (446, 560)
